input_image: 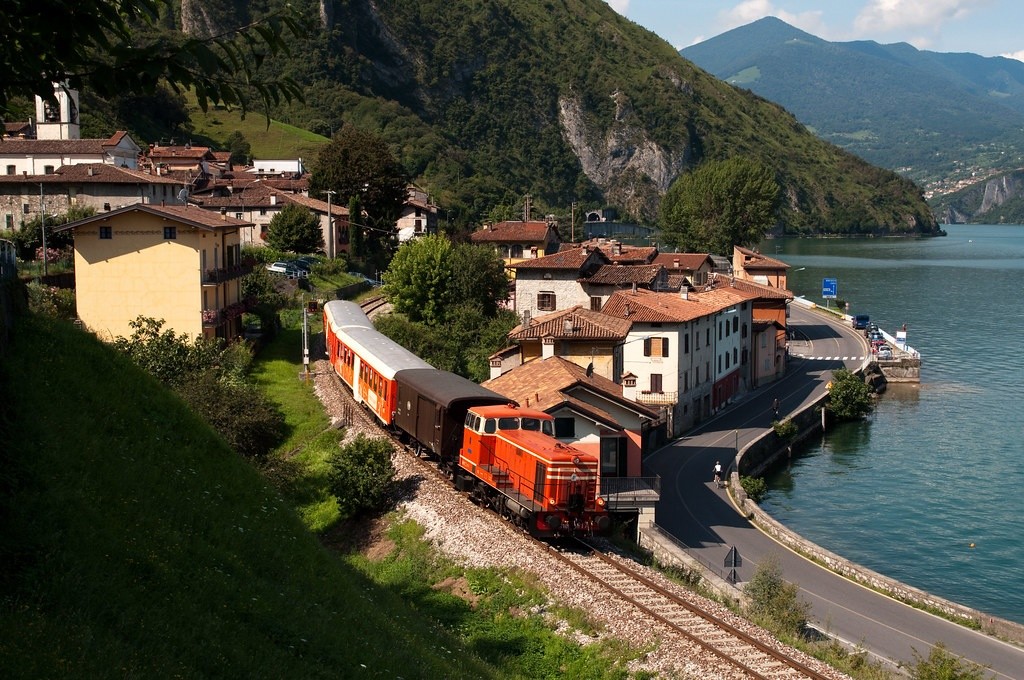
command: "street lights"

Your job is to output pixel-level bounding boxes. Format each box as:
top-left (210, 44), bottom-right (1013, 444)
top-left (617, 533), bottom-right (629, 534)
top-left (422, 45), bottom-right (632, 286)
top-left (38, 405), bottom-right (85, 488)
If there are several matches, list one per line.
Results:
top-left (242, 178), bottom-right (261, 242)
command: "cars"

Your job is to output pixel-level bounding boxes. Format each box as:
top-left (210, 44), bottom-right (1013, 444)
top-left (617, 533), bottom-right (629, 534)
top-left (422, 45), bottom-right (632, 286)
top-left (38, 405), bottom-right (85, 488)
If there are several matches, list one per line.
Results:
top-left (268, 256), bottom-right (321, 273)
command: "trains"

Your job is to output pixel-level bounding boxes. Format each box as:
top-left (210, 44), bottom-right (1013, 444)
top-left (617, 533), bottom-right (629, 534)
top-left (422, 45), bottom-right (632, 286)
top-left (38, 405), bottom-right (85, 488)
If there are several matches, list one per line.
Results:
top-left (322, 298), bottom-right (612, 545)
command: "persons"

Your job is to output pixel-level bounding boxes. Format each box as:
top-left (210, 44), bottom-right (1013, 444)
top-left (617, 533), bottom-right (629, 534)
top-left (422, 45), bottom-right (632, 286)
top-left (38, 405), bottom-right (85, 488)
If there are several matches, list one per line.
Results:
top-left (712, 461), bottom-right (722, 483)
